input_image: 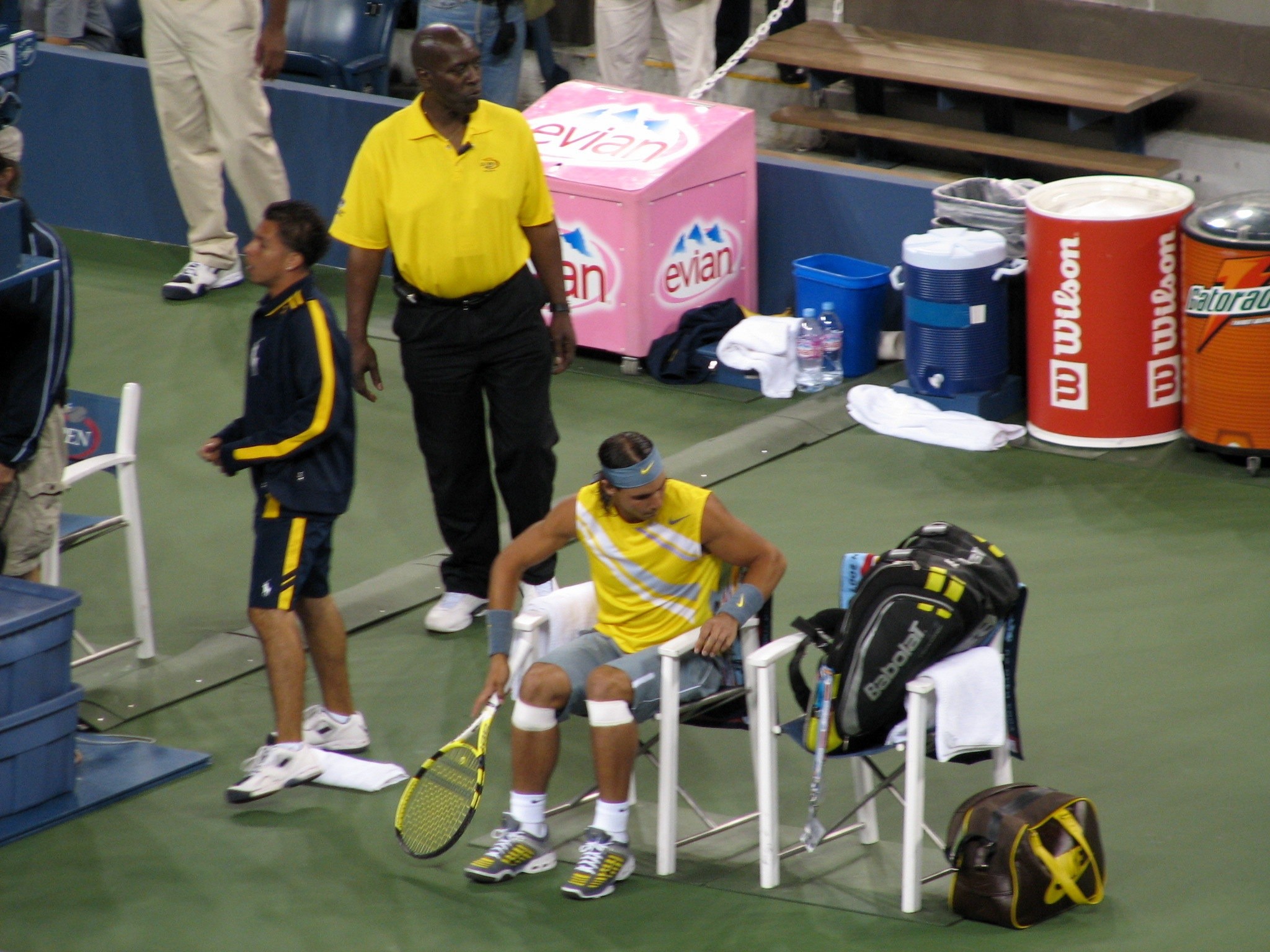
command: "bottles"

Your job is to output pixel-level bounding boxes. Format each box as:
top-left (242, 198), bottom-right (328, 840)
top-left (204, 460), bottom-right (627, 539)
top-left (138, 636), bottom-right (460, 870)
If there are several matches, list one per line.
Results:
top-left (795, 308), bottom-right (825, 393)
top-left (817, 302), bottom-right (844, 386)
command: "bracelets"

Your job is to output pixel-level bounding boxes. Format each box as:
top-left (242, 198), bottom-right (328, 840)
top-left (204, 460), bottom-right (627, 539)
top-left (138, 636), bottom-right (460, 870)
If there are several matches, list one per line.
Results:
top-left (715, 583), bottom-right (765, 628)
top-left (485, 608), bottom-right (516, 657)
top-left (550, 302), bottom-right (570, 312)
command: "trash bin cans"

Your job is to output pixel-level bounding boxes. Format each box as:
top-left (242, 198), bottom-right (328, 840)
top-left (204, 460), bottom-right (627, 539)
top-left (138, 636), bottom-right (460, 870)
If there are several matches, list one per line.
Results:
top-left (931, 174), bottom-right (1043, 263)
top-left (789, 252), bottom-right (893, 379)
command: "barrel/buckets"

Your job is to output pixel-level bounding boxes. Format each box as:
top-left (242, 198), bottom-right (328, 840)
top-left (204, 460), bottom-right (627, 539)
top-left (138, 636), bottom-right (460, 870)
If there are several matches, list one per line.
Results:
top-left (888, 227), bottom-right (1029, 395)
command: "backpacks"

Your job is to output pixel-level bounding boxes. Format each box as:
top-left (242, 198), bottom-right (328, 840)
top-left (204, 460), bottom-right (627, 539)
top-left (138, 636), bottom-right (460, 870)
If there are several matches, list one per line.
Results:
top-left (788, 520), bottom-right (1020, 752)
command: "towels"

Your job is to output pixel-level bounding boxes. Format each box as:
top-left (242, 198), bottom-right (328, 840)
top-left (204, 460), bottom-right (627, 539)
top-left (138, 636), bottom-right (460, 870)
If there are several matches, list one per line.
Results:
top-left (507, 581), bottom-right (600, 702)
top-left (299, 746), bottom-right (411, 796)
top-left (843, 383), bottom-right (1027, 454)
top-left (887, 646), bottom-right (1012, 767)
top-left (715, 310), bottom-right (803, 398)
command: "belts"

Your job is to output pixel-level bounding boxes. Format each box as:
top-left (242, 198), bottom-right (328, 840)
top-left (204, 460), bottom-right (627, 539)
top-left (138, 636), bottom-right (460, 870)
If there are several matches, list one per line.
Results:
top-left (394, 267), bottom-right (530, 312)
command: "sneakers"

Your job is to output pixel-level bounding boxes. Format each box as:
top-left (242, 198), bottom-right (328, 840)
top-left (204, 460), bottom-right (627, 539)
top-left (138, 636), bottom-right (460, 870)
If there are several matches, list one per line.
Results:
top-left (463, 812), bottom-right (559, 884)
top-left (223, 742), bottom-right (324, 804)
top-left (163, 255), bottom-right (244, 300)
top-left (267, 705), bottom-right (370, 752)
top-left (561, 825), bottom-right (636, 899)
top-left (424, 592), bottom-right (490, 632)
top-left (518, 578), bottom-right (558, 604)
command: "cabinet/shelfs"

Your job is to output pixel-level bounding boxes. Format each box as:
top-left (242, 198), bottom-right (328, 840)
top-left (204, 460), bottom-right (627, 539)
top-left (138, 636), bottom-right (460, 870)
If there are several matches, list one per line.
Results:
top-left (747, 20), bottom-right (1200, 182)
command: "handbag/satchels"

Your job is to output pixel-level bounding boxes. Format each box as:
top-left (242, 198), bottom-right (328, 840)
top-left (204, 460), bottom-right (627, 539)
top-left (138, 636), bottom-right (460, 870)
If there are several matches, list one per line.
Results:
top-left (945, 781), bottom-right (1108, 928)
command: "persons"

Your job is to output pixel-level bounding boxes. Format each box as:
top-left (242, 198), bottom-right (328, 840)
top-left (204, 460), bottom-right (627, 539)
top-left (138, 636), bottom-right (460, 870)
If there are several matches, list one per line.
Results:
top-left (135, 0), bottom-right (296, 299)
top-left (461, 432), bottom-right (790, 900)
top-left (15, 0), bottom-right (125, 55)
top-left (194, 196), bottom-right (371, 807)
top-left (323, 21), bottom-right (577, 635)
top-left (411, 0), bottom-right (804, 110)
top-left (0, 114), bottom-right (87, 772)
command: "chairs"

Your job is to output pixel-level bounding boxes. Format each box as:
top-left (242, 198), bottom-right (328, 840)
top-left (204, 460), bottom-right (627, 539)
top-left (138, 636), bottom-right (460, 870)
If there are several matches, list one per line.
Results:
top-left (508, 563), bottom-right (764, 878)
top-left (743, 553), bottom-right (1028, 918)
top-left (99, 0), bottom-right (403, 101)
top-left (38, 383), bottom-right (159, 668)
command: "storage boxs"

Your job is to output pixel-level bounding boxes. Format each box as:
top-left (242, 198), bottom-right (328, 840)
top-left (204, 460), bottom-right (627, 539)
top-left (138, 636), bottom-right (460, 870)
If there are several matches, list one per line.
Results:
top-left (0, 574), bottom-right (83, 823)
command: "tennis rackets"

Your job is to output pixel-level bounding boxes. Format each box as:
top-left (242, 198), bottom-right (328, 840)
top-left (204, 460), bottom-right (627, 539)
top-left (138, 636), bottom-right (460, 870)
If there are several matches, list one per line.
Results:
top-left (393, 638), bottom-right (535, 861)
